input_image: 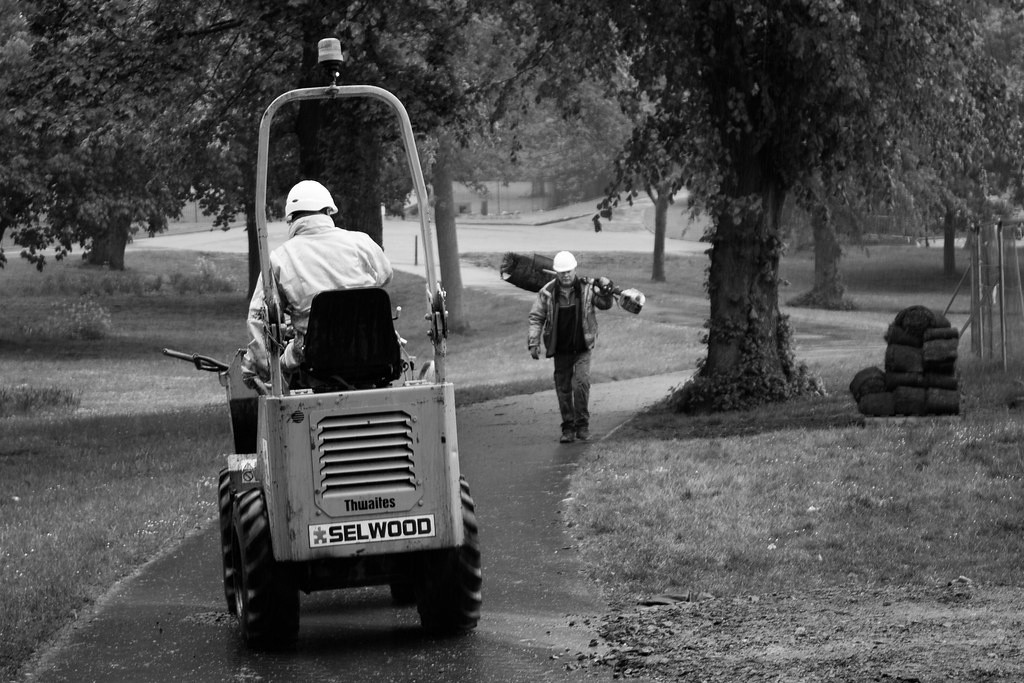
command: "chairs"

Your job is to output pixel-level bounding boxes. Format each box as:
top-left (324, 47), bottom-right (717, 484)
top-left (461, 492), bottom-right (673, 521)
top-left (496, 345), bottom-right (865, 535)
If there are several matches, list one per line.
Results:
top-left (299, 287), bottom-right (404, 393)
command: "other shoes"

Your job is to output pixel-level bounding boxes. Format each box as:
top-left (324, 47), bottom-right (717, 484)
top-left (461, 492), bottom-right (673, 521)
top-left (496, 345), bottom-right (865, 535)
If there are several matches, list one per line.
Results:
top-left (560, 433), bottom-right (575, 443)
top-left (576, 426), bottom-right (592, 441)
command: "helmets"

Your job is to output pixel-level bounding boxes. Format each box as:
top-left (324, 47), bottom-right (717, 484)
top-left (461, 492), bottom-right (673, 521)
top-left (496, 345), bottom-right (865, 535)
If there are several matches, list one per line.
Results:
top-left (553, 251), bottom-right (577, 273)
top-left (286, 180), bottom-right (337, 222)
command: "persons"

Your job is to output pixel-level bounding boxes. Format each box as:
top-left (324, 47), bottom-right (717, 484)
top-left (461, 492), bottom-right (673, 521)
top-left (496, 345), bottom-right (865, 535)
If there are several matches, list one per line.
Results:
top-left (242, 180), bottom-right (394, 388)
top-left (528, 250), bottom-right (615, 443)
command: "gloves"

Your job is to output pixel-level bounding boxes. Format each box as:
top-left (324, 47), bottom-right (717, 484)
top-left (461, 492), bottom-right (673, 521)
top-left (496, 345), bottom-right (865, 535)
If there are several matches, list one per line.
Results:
top-left (593, 276), bottom-right (613, 299)
top-left (240, 354), bottom-right (273, 389)
